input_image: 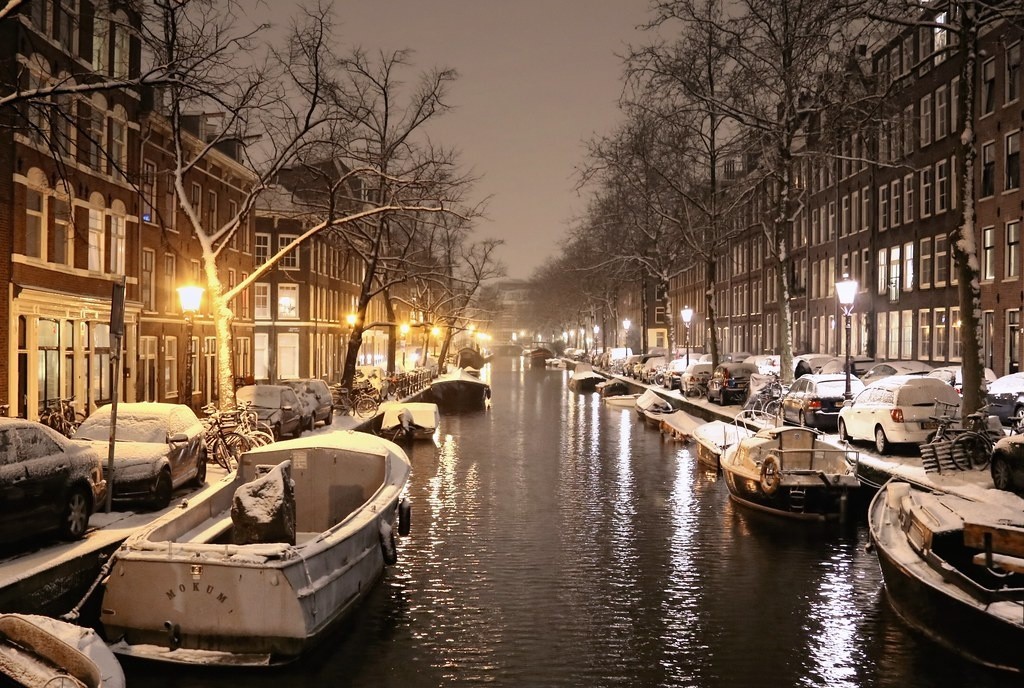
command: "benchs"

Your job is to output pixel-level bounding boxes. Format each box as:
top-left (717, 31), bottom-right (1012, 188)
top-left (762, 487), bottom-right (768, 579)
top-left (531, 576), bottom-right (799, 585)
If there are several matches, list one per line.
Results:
top-left (0, 642), bottom-right (85, 688)
top-left (963, 522), bottom-right (1024, 574)
top-left (174, 505), bottom-right (234, 544)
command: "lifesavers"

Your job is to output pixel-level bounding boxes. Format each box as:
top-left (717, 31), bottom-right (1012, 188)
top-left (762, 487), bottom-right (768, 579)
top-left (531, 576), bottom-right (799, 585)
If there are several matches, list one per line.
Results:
top-left (758, 454), bottom-right (782, 493)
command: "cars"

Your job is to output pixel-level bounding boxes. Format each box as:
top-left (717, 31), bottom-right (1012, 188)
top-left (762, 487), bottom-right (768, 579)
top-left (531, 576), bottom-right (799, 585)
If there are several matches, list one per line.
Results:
top-left (356, 355), bottom-right (446, 400)
top-left (563, 346), bottom-right (713, 395)
top-left (236, 376), bottom-right (334, 438)
top-left (706, 354), bottom-right (1024, 454)
top-left (0, 403), bottom-right (208, 553)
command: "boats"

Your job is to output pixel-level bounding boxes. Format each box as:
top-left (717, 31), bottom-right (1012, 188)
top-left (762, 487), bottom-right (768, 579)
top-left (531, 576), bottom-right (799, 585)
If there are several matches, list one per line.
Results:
top-left (381, 364), bottom-right (492, 443)
top-left (523, 341), bottom-right (605, 389)
top-left (717, 413), bottom-right (1024, 679)
top-left (596, 380), bottom-right (756, 468)
top-left (0, 430), bottom-right (410, 688)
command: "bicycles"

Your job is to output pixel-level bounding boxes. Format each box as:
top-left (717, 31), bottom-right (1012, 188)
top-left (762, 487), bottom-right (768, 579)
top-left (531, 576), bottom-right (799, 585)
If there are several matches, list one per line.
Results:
top-left (327, 379), bottom-right (382, 419)
top-left (39, 396), bottom-right (85, 438)
top-left (649, 365), bottom-right (669, 386)
top-left (929, 399), bottom-right (1024, 473)
top-left (684, 378), bottom-right (708, 400)
top-left (610, 358), bottom-right (627, 375)
top-left (199, 400), bottom-right (276, 473)
top-left (752, 377), bottom-right (790, 421)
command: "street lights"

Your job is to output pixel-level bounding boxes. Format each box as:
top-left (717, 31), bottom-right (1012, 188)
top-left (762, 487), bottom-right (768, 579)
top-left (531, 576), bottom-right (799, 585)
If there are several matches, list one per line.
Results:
top-left (177, 279), bottom-right (488, 402)
top-left (562, 273), bottom-right (855, 400)
